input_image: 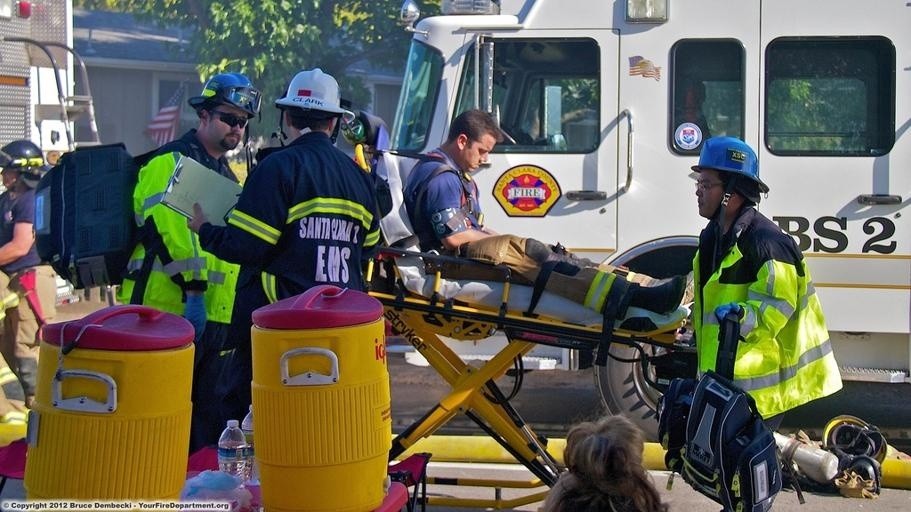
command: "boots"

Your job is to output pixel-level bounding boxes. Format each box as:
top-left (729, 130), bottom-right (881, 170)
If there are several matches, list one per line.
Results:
top-left (630, 274), bottom-right (686, 316)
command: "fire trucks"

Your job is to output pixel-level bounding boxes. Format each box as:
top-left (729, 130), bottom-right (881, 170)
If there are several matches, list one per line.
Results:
top-left (380, 0), bottom-right (908, 445)
top-left (0, 0), bottom-right (107, 312)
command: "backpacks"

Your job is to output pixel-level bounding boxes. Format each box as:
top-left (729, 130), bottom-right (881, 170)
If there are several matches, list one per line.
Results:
top-left (655, 313), bottom-right (806, 512)
top-left (34, 140), bottom-right (190, 304)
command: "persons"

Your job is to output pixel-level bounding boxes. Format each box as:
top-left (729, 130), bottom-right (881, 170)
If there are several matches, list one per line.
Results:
top-left (117, 72), bottom-right (262, 452)
top-left (403, 109), bottom-right (695, 314)
top-left (688, 135), bottom-right (844, 432)
top-left (186, 66), bottom-right (386, 442)
top-left (0, 139), bottom-right (59, 409)
top-left (529, 410), bottom-right (674, 511)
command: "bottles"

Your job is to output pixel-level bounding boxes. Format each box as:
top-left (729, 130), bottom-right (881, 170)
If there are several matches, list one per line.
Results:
top-left (217, 419), bottom-right (247, 494)
top-left (241, 405), bottom-right (261, 485)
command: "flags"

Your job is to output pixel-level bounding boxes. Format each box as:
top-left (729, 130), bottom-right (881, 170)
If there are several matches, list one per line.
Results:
top-left (145, 84), bottom-right (185, 146)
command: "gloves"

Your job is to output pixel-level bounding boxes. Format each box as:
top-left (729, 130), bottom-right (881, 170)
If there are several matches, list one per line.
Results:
top-left (184, 293), bottom-right (207, 342)
top-left (714, 302), bottom-right (739, 321)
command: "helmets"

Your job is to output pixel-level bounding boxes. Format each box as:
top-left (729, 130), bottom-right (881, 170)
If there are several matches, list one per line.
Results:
top-left (0, 139), bottom-right (49, 189)
top-left (275, 68), bottom-right (354, 120)
top-left (690, 136), bottom-right (769, 194)
top-left (187, 73), bottom-right (264, 119)
top-left (822, 413), bottom-right (888, 465)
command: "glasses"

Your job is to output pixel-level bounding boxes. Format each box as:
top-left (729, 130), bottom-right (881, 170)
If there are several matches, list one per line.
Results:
top-left (210, 110), bottom-right (249, 128)
top-left (695, 181), bottom-right (724, 191)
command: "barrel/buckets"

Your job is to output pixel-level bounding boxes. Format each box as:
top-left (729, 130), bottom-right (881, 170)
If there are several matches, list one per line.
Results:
top-left (21, 303), bottom-right (198, 512)
top-left (246, 282), bottom-right (392, 512)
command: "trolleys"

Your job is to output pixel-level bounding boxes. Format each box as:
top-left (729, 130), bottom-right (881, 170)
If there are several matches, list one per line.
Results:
top-left (336, 110), bottom-right (693, 511)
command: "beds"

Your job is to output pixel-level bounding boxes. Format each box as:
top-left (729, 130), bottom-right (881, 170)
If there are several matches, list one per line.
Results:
top-left (365, 244), bottom-right (696, 512)
